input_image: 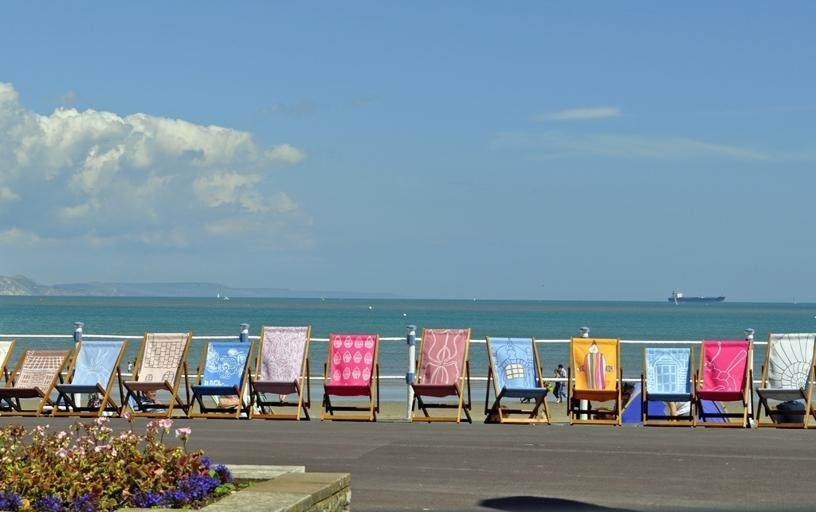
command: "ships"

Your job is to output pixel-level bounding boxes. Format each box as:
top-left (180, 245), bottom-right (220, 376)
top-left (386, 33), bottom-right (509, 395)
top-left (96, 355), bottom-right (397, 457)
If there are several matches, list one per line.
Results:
top-left (665, 291), bottom-right (725, 305)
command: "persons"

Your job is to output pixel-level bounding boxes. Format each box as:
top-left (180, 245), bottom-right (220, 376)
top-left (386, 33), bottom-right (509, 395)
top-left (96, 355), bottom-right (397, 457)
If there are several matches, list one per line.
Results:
top-left (133, 357), bottom-right (137, 366)
top-left (558, 364), bottom-right (568, 404)
top-left (217, 394), bottom-right (240, 409)
top-left (0, 399), bottom-right (12, 411)
top-left (662, 401), bottom-right (691, 422)
top-left (570, 385), bottom-right (591, 419)
top-left (126, 362), bottom-right (133, 373)
top-left (279, 394), bottom-right (286, 402)
top-left (140, 390), bottom-right (168, 413)
top-left (553, 368), bottom-right (561, 402)
top-left (776, 401), bottom-right (805, 423)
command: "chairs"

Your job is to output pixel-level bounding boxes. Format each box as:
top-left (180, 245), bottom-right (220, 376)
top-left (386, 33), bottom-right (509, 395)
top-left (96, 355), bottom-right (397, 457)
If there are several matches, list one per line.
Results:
top-left (569, 336), bottom-right (622, 426)
top-left (120, 332), bottom-right (192, 418)
top-left (642, 346), bottom-right (696, 428)
top-left (696, 339), bottom-right (754, 428)
top-left (50, 338), bottom-right (128, 417)
top-left (0, 339), bottom-right (15, 417)
top-left (0, 349), bottom-right (71, 417)
top-left (410, 327), bottom-right (472, 424)
top-left (188, 340), bottom-right (254, 419)
top-left (485, 335), bottom-right (551, 425)
top-left (250, 324), bottom-right (312, 420)
top-left (320, 333), bottom-right (380, 421)
top-left (755, 333), bottom-right (816, 429)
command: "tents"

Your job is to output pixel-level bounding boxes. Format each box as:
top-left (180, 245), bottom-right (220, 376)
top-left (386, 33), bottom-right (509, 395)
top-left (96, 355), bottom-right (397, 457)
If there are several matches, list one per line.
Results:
top-left (621, 383), bottom-right (730, 426)
